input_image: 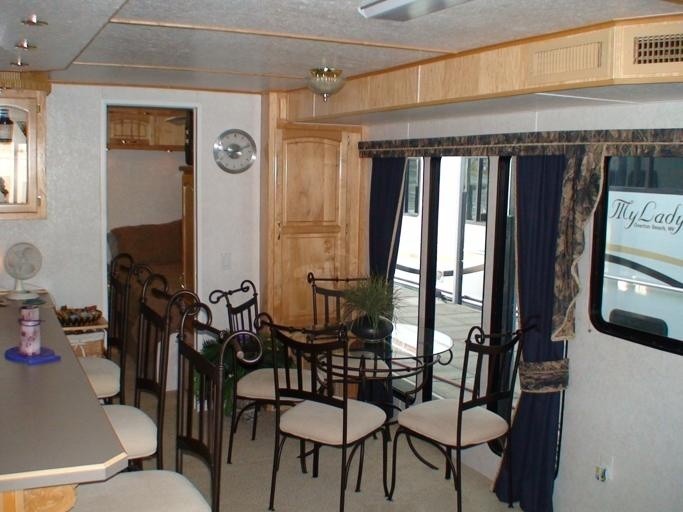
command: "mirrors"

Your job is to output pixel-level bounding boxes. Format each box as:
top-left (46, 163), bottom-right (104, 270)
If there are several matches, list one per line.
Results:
top-left (0, 96), bottom-right (36, 213)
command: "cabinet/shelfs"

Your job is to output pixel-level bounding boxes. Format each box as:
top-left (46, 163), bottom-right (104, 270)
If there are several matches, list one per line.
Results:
top-left (0, 76), bottom-right (51, 219)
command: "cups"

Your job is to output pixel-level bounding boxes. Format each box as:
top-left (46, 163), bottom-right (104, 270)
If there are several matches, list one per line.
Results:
top-left (19, 305), bottom-right (39, 325)
top-left (19, 318), bottom-right (44, 356)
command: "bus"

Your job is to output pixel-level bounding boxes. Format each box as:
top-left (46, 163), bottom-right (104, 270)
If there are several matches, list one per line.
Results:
top-left (390, 153), bottom-right (682, 346)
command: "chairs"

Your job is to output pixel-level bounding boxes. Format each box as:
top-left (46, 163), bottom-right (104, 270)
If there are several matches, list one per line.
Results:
top-left (64, 249), bottom-right (523, 511)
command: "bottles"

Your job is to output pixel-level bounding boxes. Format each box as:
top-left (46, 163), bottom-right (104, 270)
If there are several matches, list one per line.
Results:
top-left (0, 178), bottom-right (11, 205)
top-left (0, 108), bottom-right (14, 144)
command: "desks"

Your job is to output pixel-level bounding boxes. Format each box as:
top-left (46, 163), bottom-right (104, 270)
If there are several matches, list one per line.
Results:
top-left (1, 286), bottom-right (130, 511)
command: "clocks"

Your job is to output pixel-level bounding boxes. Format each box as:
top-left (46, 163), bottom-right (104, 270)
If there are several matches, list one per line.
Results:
top-left (212, 128), bottom-right (258, 175)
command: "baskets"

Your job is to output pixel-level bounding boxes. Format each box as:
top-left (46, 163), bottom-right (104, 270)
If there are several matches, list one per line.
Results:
top-left (55, 306), bottom-right (102, 327)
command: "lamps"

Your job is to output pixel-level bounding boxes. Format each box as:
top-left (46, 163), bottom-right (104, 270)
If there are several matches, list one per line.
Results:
top-left (355, 0), bottom-right (473, 23)
top-left (307, 67), bottom-right (345, 102)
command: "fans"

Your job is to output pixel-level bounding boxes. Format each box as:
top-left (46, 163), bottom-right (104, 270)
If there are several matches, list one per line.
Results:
top-left (3, 243), bottom-right (42, 301)
